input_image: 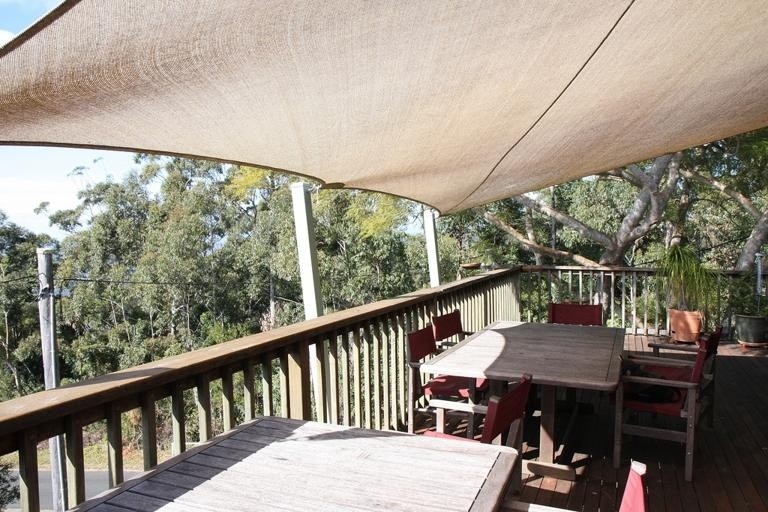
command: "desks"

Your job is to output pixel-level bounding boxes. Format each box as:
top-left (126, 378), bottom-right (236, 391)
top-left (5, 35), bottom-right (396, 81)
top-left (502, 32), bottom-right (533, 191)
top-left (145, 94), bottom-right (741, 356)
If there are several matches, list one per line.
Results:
top-left (66, 416), bottom-right (519, 512)
top-left (419, 320), bottom-right (628, 481)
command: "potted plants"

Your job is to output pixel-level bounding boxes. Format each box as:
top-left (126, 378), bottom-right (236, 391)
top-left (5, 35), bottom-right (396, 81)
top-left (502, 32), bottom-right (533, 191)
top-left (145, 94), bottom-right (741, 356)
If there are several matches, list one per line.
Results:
top-left (722, 269), bottom-right (768, 347)
top-left (656, 245), bottom-right (714, 342)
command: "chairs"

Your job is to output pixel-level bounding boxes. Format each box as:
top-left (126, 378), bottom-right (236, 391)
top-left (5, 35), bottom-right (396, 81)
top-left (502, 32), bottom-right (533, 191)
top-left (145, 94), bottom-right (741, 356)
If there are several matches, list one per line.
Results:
top-left (549, 302), bottom-right (602, 327)
top-left (621, 327), bottom-right (721, 483)
top-left (421, 372), bottom-right (534, 503)
top-left (408, 309), bottom-right (519, 440)
